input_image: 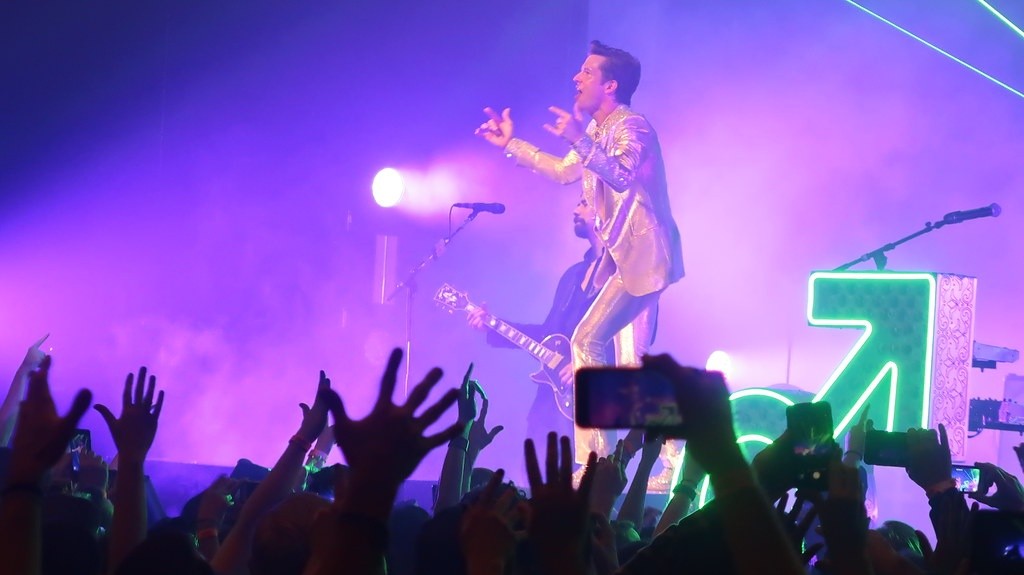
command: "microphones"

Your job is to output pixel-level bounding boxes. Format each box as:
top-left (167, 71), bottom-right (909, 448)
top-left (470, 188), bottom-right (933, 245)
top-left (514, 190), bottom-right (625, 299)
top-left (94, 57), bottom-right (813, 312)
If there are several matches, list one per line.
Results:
top-left (453, 202), bottom-right (507, 214)
top-left (942, 202), bottom-right (1003, 225)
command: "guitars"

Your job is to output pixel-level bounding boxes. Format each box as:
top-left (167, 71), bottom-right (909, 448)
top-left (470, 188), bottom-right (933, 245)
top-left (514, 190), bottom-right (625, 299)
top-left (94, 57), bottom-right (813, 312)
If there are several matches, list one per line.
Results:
top-left (434, 282), bottom-right (574, 421)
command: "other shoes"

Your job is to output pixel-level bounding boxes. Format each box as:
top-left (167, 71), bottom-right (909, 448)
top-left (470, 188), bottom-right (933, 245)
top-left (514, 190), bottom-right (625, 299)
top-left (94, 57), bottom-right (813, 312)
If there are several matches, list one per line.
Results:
top-left (648, 468), bottom-right (673, 492)
top-left (572, 465), bottom-right (585, 487)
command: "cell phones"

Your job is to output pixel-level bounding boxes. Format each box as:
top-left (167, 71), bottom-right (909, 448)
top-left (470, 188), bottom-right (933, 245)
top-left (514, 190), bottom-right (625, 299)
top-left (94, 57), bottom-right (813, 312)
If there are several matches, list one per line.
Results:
top-left (863, 430), bottom-right (915, 468)
top-left (786, 401), bottom-right (833, 487)
top-left (951, 465), bottom-right (989, 495)
top-left (573, 365), bottom-right (686, 428)
top-left (70, 428), bottom-right (92, 472)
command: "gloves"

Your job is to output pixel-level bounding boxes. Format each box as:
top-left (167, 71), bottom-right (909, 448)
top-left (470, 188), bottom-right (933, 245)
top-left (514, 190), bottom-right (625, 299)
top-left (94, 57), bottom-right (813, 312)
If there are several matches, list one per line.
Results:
top-left (903, 422), bottom-right (954, 490)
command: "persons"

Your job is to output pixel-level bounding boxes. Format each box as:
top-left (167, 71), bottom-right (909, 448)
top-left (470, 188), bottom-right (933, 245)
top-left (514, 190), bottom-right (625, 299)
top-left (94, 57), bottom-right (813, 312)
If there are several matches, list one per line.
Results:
top-left (468, 190), bottom-right (620, 484)
top-left (475, 39), bottom-right (685, 495)
top-left (1, 331), bottom-right (1024, 575)
